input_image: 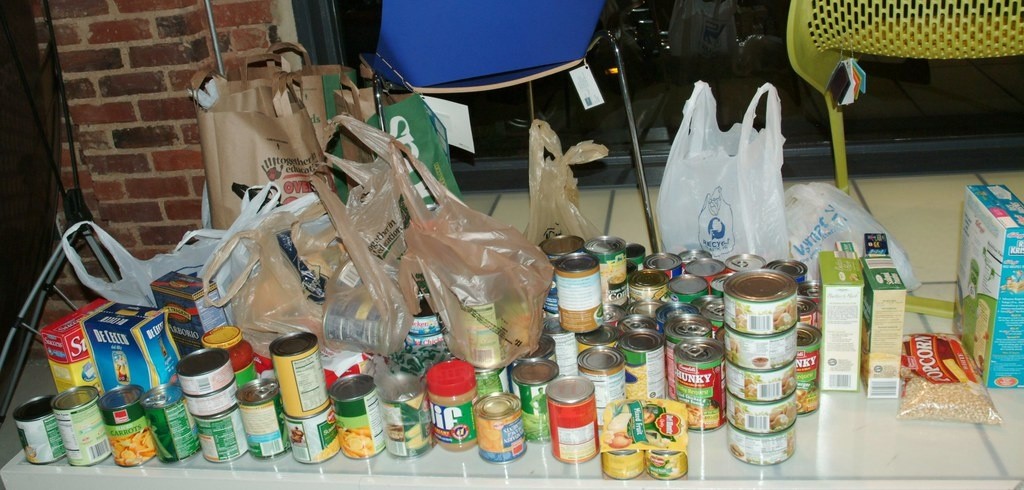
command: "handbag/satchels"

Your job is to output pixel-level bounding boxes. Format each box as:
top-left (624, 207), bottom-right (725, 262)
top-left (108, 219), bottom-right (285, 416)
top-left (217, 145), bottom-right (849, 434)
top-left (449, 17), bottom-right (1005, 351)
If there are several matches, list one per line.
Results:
top-left (655, 80), bottom-right (787, 262)
top-left (521, 119), bottom-right (610, 243)
top-left (785, 181), bottom-right (921, 294)
top-left (666, 0), bottom-right (738, 61)
top-left (61, 43), bottom-right (556, 370)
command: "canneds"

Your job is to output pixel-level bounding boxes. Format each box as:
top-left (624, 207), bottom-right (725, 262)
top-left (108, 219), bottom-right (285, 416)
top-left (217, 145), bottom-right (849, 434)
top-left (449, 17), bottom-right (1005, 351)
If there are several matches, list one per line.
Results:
top-left (10, 233), bottom-right (824, 481)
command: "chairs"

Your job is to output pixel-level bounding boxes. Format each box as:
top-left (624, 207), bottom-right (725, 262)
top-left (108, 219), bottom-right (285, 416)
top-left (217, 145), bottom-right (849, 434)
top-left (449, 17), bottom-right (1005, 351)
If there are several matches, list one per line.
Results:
top-left (361, 0), bottom-right (1024, 253)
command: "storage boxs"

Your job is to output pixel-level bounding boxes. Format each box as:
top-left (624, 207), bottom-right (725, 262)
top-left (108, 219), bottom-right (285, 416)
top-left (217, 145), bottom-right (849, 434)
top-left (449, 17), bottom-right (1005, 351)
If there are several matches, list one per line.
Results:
top-left (38, 269), bottom-right (237, 408)
top-left (819, 185), bottom-right (1024, 399)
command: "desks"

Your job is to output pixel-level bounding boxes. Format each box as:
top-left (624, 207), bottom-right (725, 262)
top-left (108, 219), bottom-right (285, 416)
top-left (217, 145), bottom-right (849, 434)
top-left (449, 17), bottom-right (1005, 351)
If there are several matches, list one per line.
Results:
top-left (0, 280), bottom-right (1024, 490)
